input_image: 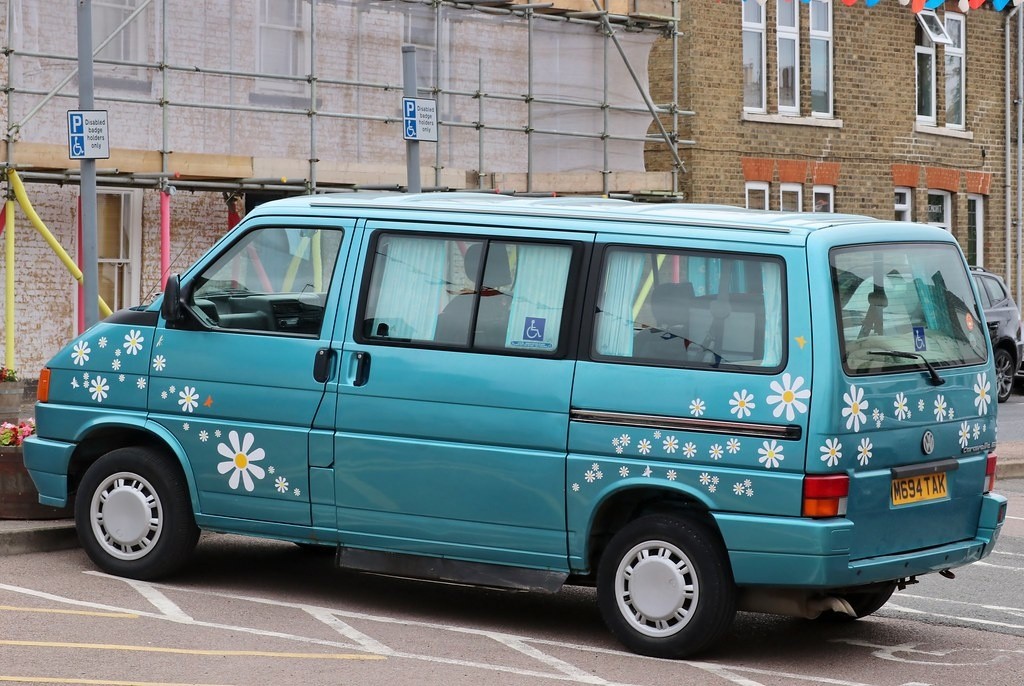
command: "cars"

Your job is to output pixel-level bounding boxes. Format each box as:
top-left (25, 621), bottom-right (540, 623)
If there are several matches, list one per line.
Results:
top-left (965, 267), bottom-right (1018, 404)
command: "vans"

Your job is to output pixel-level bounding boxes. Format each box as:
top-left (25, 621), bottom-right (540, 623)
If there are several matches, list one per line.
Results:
top-left (19, 189), bottom-right (1009, 658)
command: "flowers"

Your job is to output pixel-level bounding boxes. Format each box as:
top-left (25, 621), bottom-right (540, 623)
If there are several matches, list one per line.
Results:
top-left (0, 417), bottom-right (36, 446)
top-left (0, 367), bottom-right (17, 382)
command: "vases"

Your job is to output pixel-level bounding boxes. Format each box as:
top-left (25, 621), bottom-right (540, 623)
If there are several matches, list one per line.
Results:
top-left (0, 382), bottom-right (25, 426)
top-left (0, 446), bottom-right (72, 519)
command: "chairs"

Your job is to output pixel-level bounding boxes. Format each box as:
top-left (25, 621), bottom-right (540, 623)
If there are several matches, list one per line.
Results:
top-left (632, 281), bottom-right (694, 361)
top-left (434, 243), bottom-right (513, 343)
